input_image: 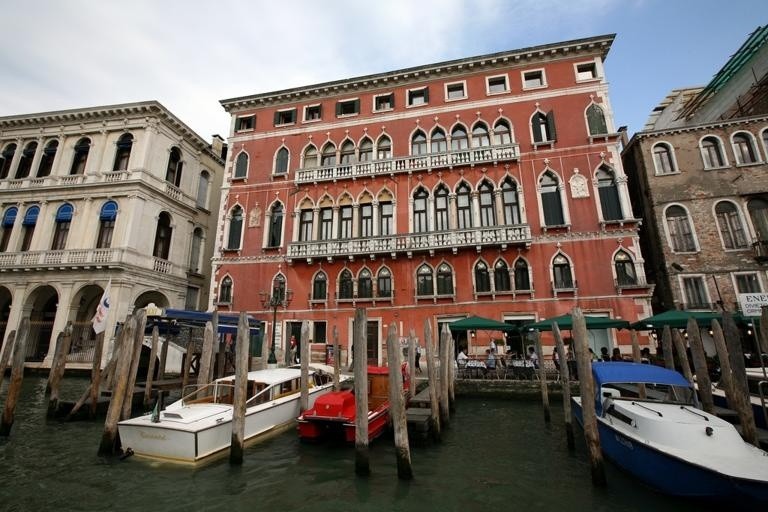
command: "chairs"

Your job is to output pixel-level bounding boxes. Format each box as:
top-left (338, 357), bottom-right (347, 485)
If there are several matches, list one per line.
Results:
top-left (456, 349), bottom-right (578, 380)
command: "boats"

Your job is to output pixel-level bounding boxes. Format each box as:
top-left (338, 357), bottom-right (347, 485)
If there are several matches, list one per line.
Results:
top-left (693, 364), bottom-right (768, 430)
top-left (295, 363), bottom-right (411, 450)
top-left (114, 361), bottom-right (345, 471)
top-left (569, 360), bottom-right (768, 509)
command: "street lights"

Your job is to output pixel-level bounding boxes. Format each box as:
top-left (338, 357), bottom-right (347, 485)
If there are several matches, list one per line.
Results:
top-left (260, 286), bottom-right (294, 363)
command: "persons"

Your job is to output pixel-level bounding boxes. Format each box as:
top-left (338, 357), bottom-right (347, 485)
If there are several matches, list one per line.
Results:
top-left (553, 344), bottom-right (655, 371)
top-left (457, 348), bottom-right (472, 367)
top-left (288, 334), bottom-right (298, 366)
top-left (490, 348), bottom-right (504, 368)
top-left (347, 345), bottom-right (354, 372)
top-left (528, 346), bottom-right (539, 369)
top-left (480, 349), bottom-right (495, 375)
top-left (489, 338), bottom-right (496, 349)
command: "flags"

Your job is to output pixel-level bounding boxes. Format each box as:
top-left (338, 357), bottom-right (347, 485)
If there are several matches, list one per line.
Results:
top-left (92, 276), bottom-right (112, 336)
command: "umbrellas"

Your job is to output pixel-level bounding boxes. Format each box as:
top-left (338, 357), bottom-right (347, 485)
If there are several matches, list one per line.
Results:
top-left (439, 314), bottom-right (517, 363)
top-left (631, 308), bottom-right (743, 334)
top-left (519, 314), bottom-right (631, 358)
